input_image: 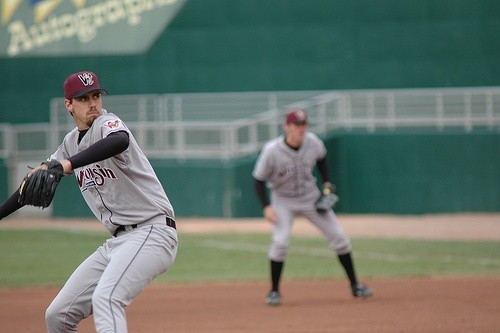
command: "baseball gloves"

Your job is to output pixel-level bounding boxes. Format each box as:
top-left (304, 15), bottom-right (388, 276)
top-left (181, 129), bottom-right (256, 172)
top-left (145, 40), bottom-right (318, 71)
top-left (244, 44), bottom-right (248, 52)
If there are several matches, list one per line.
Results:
top-left (17, 159), bottom-right (64, 211)
top-left (315, 182), bottom-right (340, 214)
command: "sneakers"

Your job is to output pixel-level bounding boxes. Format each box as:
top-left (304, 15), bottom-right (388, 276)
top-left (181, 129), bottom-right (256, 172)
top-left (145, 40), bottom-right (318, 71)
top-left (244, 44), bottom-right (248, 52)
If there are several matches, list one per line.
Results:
top-left (266, 289), bottom-right (281, 306)
top-left (351, 283), bottom-right (373, 297)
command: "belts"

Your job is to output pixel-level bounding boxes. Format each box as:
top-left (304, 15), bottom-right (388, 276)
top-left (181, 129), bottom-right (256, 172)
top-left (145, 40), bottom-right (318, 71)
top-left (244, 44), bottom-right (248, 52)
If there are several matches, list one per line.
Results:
top-left (114, 216), bottom-right (178, 236)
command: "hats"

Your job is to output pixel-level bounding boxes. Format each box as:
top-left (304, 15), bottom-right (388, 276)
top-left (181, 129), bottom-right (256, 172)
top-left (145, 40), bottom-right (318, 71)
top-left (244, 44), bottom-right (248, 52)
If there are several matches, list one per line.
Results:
top-left (63, 71), bottom-right (108, 97)
top-left (285, 109), bottom-right (307, 125)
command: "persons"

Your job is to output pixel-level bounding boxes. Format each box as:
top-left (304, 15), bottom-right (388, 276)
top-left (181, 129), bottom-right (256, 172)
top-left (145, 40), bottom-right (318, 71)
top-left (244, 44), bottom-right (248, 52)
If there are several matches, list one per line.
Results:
top-left (250, 109), bottom-right (377, 307)
top-left (0, 71), bottom-right (179, 333)
